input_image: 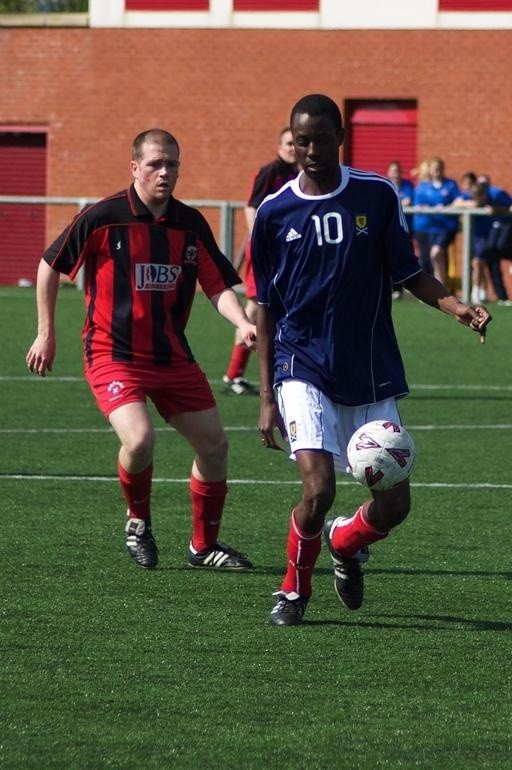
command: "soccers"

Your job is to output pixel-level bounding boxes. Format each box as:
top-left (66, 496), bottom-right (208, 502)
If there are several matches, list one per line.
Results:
top-left (347, 419), bottom-right (419, 488)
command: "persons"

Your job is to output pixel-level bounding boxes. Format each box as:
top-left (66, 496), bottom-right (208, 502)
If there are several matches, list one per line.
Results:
top-left (386, 157), bottom-right (512, 307)
top-left (250, 94), bottom-right (493, 628)
top-left (26, 129), bottom-right (256, 572)
top-left (221, 126), bottom-right (302, 397)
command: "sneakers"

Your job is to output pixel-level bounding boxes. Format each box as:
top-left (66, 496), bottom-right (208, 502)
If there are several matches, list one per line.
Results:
top-left (270, 590), bottom-right (310, 626)
top-left (218, 374), bottom-right (259, 396)
top-left (323, 516), bottom-right (369, 610)
top-left (187, 539), bottom-right (252, 570)
top-left (124, 507), bottom-right (160, 569)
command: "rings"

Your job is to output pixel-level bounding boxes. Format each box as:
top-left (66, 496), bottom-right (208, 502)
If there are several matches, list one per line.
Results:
top-left (261, 439), bottom-right (264, 442)
